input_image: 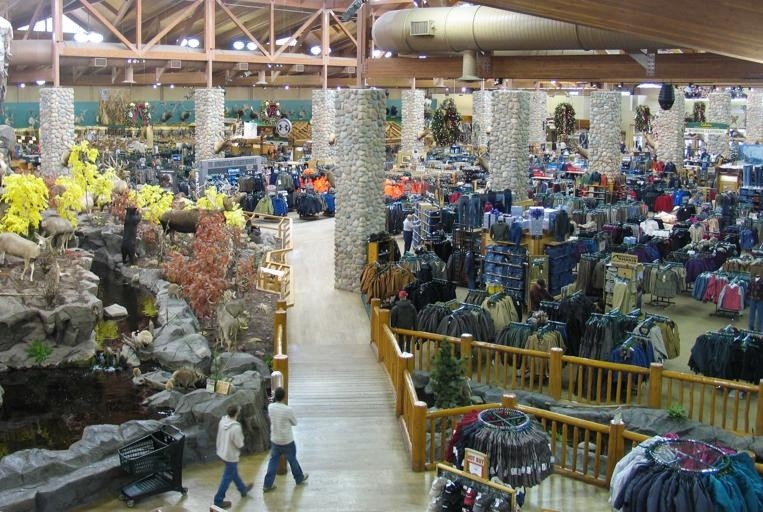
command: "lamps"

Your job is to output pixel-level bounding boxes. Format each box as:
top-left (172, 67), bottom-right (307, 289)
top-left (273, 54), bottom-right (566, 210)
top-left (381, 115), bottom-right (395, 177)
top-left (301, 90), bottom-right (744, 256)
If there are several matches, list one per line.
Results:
top-left (233, 40), bottom-right (257, 51)
top-left (266, 37), bottom-right (300, 48)
top-left (73, 9), bottom-right (104, 43)
top-left (175, 37), bottom-right (200, 48)
top-left (311, 44), bottom-right (332, 55)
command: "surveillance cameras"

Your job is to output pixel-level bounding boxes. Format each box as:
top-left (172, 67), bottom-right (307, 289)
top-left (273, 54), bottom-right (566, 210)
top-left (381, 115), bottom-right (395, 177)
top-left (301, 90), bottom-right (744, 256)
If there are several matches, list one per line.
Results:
top-left (658, 85), bottom-right (675, 111)
top-left (338, 0), bottom-right (362, 22)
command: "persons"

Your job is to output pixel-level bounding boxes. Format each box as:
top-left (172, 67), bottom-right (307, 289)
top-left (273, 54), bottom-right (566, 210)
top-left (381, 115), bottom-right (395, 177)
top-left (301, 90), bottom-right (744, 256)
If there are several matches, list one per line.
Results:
top-left (211, 404), bottom-right (253, 509)
top-left (530, 280), bottom-right (551, 309)
top-left (262, 386), bottom-right (309, 492)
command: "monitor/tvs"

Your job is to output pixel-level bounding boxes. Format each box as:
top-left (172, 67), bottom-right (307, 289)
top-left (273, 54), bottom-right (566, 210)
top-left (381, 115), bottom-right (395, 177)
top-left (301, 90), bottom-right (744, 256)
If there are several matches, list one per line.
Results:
top-left (227, 168), bottom-right (241, 173)
top-left (173, 155), bottom-right (181, 161)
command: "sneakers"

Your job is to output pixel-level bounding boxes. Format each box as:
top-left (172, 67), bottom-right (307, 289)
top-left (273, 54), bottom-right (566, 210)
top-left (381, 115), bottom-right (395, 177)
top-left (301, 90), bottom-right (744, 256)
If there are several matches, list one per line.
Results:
top-left (220, 501), bottom-right (231, 508)
top-left (242, 483), bottom-right (253, 497)
top-left (302, 473), bottom-right (307, 480)
top-left (263, 486), bottom-right (276, 491)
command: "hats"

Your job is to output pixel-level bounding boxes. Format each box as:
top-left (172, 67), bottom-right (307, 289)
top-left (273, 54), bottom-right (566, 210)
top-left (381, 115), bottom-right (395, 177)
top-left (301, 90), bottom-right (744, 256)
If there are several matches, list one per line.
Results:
top-left (399, 290), bottom-right (408, 297)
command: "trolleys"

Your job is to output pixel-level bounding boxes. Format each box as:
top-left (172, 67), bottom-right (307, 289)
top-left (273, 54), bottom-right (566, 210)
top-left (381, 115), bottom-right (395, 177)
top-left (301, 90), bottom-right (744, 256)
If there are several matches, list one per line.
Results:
top-left (114, 422), bottom-right (190, 509)
